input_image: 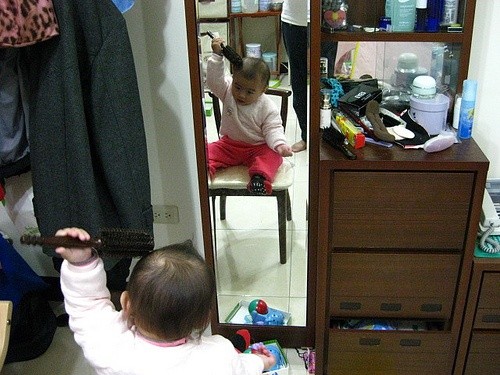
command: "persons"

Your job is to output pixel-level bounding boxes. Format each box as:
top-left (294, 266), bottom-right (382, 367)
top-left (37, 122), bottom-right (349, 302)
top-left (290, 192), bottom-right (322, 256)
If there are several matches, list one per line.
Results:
top-left (53, 227), bottom-right (276, 375)
top-left (280, 0), bottom-right (307, 153)
top-left (206, 36), bottom-right (293, 196)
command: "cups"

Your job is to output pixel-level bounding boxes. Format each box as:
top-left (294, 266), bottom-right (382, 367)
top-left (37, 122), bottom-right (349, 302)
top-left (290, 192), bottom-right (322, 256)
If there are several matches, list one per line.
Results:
top-left (245, 43), bottom-right (261, 59)
top-left (230, 0), bottom-right (270, 14)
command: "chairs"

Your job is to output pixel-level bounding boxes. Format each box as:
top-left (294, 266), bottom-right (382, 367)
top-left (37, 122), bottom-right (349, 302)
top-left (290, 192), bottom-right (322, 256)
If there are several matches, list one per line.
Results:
top-left (207, 87), bottom-right (294, 265)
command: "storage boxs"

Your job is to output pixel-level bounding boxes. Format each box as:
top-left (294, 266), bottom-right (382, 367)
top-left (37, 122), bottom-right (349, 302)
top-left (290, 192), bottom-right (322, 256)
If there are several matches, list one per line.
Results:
top-left (335, 115), bottom-right (365, 149)
top-left (409, 93), bottom-right (450, 136)
top-left (395, 68), bottom-right (428, 90)
top-left (224, 299), bottom-right (292, 325)
top-left (244, 339), bottom-right (290, 375)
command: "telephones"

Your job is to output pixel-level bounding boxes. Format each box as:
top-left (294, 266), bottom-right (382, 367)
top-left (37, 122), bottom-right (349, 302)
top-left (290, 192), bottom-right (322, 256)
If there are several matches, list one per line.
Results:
top-left (476, 177), bottom-right (500, 237)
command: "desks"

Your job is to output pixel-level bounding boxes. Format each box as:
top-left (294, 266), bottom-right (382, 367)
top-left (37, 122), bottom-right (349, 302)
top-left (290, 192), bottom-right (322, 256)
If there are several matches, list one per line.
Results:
top-left (228, 11), bottom-right (282, 76)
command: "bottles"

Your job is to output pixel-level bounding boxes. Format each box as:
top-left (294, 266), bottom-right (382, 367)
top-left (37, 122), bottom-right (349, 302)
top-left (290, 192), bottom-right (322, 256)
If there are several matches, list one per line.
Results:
top-left (430, 46), bottom-right (444, 85)
top-left (385, 0), bottom-right (459, 32)
top-left (452, 79), bottom-right (478, 139)
top-left (320, 57), bottom-right (328, 79)
top-left (319, 92), bottom-right (333, 129)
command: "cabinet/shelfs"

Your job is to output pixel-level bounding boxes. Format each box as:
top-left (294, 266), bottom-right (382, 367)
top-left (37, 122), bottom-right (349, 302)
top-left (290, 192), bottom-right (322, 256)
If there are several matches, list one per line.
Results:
top-left (452, 257), bottom-right (500, 375)
top-left (320, 0), bottom-right (491, 375)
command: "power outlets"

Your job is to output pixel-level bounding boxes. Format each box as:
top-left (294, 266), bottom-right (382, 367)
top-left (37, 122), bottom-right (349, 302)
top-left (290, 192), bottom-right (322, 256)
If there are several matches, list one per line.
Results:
top-left (152, 204), bottom-right (180, 224)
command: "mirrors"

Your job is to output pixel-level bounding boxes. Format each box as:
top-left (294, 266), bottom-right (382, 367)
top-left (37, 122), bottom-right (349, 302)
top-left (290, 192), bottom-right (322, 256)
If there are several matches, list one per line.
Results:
top-left (184, 0), bottom-right (320, 351)
top-left (334, 41), bottom-right (462, 106)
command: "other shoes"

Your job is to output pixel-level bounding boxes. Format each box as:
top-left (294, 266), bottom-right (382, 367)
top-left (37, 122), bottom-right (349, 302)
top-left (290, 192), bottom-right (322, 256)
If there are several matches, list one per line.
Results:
top-left (291, 140), bottom-right (306, 152)
top-left (229, 329), bottom-right (250, 352)
top-left (247, 173), bottom-right (272, 196)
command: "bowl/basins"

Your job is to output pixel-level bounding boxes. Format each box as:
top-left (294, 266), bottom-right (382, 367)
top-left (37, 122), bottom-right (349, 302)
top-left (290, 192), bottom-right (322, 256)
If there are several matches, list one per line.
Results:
top-left (268, 2), bottom-right (282, 12)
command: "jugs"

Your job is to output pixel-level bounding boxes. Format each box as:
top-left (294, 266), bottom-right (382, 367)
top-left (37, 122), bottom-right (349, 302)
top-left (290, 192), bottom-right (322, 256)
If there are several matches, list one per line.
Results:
top-left (262, 51), bottom-right (277, 79)
